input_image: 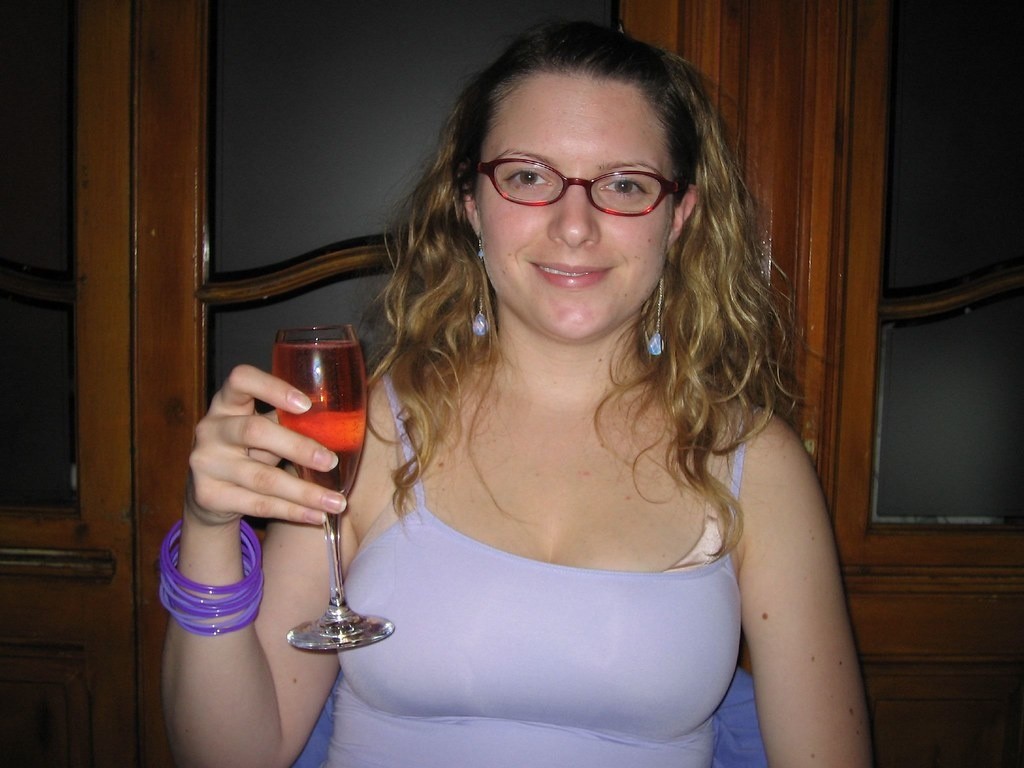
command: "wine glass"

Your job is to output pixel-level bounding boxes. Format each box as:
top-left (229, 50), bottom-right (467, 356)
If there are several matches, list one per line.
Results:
top-left (272, 325), bottom-right (395, 650)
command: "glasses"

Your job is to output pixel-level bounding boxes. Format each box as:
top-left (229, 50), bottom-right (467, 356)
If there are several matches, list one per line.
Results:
top-left (480, 157), bottom-right (679, 219)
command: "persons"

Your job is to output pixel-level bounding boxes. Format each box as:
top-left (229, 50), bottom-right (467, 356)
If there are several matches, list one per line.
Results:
top-left (159, 18), bottom-right (871, 768)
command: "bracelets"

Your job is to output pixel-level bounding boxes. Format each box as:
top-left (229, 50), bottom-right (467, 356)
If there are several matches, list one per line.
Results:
top-left (158, 518), bottom-right (264, 637)
